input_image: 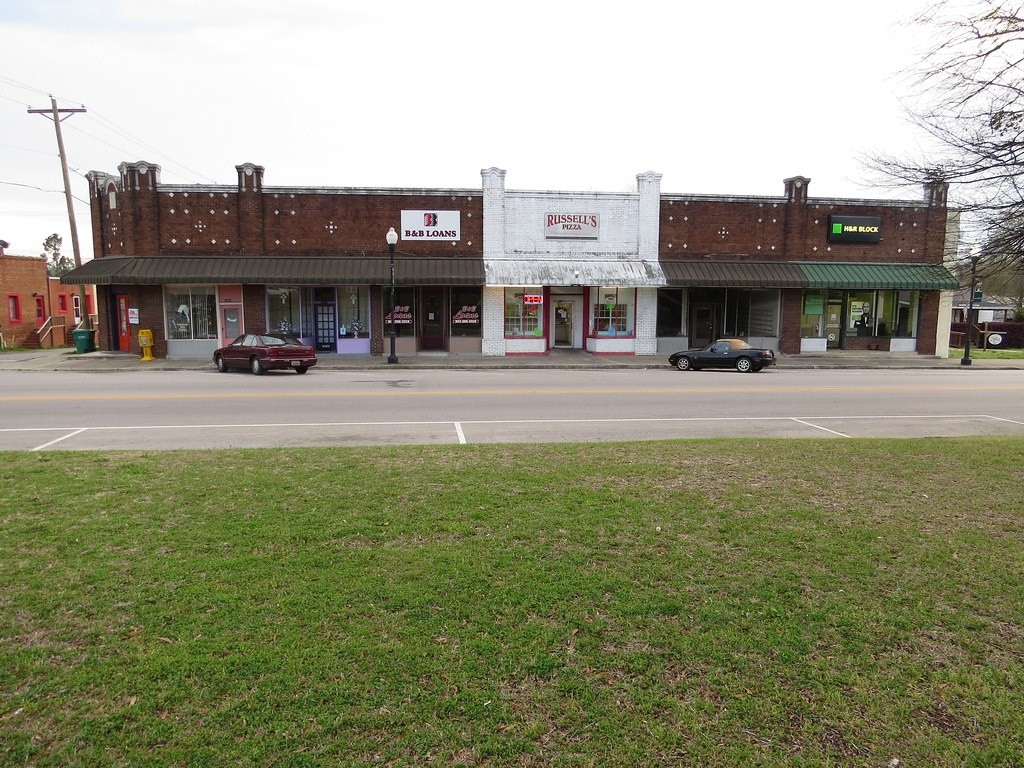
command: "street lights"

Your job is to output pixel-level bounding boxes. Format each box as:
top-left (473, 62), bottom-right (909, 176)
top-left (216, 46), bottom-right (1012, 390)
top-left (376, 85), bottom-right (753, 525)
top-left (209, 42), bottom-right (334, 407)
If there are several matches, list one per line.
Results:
top-left (385, 227), bottom-right (398, 364)
top-left (961, 242), bottom-right (981, 365)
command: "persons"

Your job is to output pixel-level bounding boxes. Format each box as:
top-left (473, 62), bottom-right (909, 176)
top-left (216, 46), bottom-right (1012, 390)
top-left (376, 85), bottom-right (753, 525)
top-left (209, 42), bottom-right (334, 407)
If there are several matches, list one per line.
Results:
top-left (854, 303), bottom-right (870, 328)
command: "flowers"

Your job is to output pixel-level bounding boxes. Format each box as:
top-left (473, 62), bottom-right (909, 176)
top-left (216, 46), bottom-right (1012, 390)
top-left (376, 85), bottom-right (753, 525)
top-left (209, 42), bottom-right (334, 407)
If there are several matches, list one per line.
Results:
top-left (277, 319), bottom-right (295, 333)
top-left (349, 320), bottom-right (363, 333)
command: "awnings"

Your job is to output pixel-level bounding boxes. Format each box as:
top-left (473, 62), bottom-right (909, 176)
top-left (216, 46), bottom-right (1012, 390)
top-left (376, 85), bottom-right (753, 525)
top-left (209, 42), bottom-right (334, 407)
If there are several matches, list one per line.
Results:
top-left (483, 260), bottom-right (666, 287)
top-left (799, 264), bottom-right (960, 290)
top-left (659, 261), bottom-right (808, 288)
top-left (60, 258), bottom-right (486, 286)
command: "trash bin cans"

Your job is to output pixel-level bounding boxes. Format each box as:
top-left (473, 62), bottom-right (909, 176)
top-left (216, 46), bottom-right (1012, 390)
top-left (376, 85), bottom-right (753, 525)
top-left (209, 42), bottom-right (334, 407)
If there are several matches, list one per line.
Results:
top-left (72, 328), bottom-right (95, 354)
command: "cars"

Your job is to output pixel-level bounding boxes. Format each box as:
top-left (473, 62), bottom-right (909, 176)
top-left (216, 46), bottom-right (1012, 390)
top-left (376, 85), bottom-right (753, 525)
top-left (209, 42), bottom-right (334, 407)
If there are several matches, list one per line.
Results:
top-left (168, 311), bottom-right (190, 332)
top-left (668, 339), bottom-right (777, 373)
top-left (212, 333), bottom-right (317, 375)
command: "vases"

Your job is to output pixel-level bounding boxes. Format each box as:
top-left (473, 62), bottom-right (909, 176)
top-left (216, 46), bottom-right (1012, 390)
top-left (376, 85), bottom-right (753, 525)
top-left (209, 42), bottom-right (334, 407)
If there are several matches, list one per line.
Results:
top-left (354, 332), bottom-right (358, 339)
top-left (281, 330), bottom-right (287, 335)
